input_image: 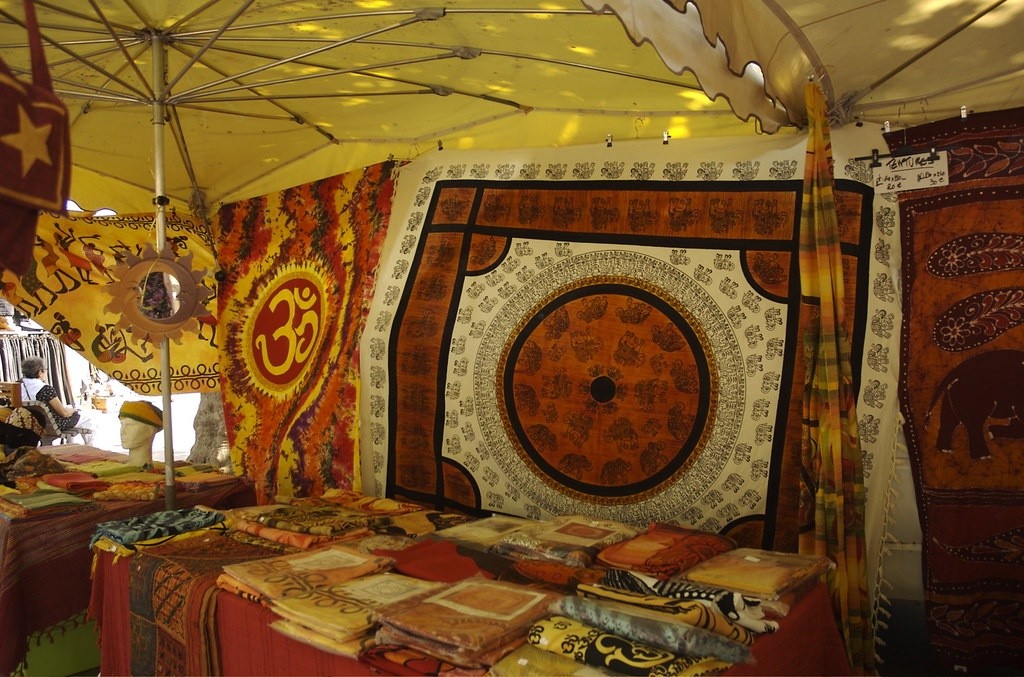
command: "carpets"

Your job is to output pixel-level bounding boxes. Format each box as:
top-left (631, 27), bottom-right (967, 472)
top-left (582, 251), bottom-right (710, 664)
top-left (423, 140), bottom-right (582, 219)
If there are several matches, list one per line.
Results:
top-left (0, 104), bottom-right (1024, 677)
top-left (387, 138), bottom-right (443, 169)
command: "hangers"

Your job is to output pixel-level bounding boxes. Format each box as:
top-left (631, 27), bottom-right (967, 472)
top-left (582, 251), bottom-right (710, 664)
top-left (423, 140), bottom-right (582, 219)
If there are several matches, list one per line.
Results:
top-left (854, 123), bottom-right (963, 168)
top-left (171, 197), bottom-right (225, 215)
top-left (606, 118), bottom-right (672, 146)
top-left (880, 97), bottom-right (975, 135)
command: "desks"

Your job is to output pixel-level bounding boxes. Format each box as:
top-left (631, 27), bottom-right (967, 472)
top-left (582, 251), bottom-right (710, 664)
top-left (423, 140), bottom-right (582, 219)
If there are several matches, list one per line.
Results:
top-left (88, 504), bottom-right (851, 677)
top-left (0, 440), bottom-right (256, 677)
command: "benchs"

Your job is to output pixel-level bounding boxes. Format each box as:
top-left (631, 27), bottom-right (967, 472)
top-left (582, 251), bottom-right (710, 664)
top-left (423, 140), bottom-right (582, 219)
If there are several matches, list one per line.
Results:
top-left (23, 400), bottom-right (92, 447)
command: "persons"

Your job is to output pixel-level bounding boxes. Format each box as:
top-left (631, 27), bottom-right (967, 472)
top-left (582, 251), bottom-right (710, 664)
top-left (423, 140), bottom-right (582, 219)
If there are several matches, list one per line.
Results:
top-left (15, 356), bottom-right (82, 431)
top-left (118, 401), bottom-right (165, 465)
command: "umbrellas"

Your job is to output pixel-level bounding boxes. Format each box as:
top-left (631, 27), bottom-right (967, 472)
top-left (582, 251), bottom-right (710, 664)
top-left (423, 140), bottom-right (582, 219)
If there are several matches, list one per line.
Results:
top-left (0, 1), bottom-right (1024, 511)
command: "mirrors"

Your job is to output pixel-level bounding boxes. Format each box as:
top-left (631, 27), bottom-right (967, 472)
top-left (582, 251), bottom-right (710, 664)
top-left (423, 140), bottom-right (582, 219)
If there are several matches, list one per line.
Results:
top-left (100, 245), bottom-right (214, 349)
